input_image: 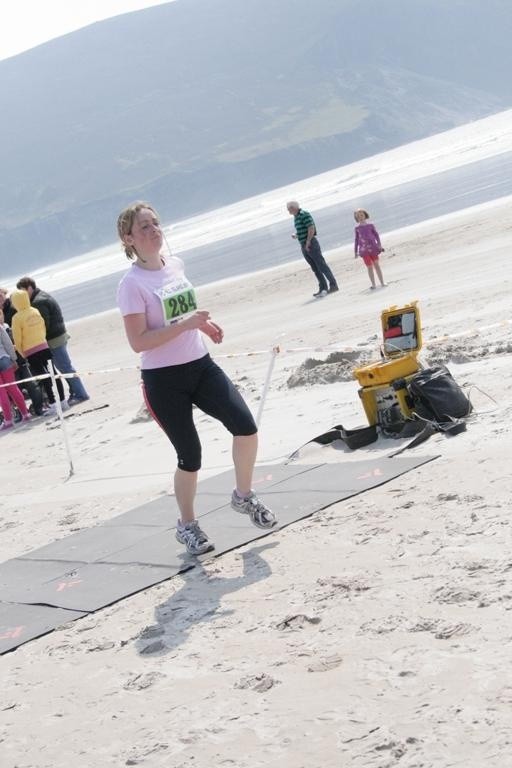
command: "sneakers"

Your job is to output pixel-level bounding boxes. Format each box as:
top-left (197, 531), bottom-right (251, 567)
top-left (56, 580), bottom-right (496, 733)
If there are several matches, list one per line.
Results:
top-left (68, 398), bottom-right (81, 406)
top-left (0, 422), bottom-right (14, 431)
top-left (231, 489), bottom-right (278, 529)
top-left (175, 519), bottom-right (215, 555)
top-left (21, 416), bottom-right (32, 423)
top-left (314, 288), bottom-right (338, 297)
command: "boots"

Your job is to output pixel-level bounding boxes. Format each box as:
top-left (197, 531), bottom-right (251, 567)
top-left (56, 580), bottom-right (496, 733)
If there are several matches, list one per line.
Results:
top-left (43, 399), bottom-right (71, 416)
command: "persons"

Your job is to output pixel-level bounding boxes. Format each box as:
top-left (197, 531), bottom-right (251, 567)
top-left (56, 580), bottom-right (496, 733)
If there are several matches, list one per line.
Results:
top-left (16, 276), bottom-right (90, 408)
top-left (116, 201), bottom-right (279, 557)
top-left (9, 289), bottom-right (68, 416)
top-left (286, 200), bottom-right (340, 298)
top-left (352, 208), bottom-right (388, 290)
top-left (0, 309), bottom-right (43, 418)
top-left (0, 287), bottom-right (35, 400)
top-left (0, 324), bottom-right (33, 431)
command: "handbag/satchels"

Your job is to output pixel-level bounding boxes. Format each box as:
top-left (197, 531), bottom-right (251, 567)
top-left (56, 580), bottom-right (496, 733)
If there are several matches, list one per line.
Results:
top-left (409, 366), bottom-right (473, 427)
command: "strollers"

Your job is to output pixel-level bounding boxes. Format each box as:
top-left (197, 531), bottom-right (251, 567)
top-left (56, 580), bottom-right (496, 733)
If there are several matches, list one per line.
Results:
top-left (8, 349), bottom-right (52, 424)
